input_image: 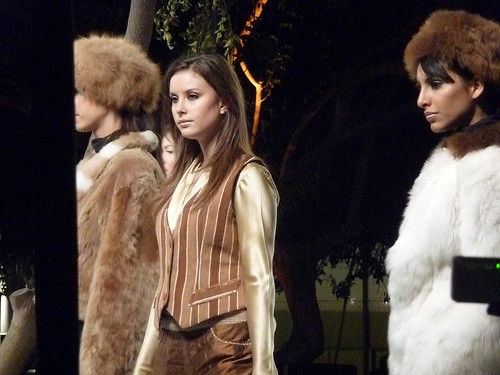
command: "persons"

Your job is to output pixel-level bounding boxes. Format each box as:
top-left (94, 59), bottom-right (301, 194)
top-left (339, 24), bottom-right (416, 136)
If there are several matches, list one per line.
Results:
top-left (74, 39), bottom-right (163, 375)
top-left (385, 11), bottom-right (500, 375)
top-left (135, 56), bottom-right (280, 375)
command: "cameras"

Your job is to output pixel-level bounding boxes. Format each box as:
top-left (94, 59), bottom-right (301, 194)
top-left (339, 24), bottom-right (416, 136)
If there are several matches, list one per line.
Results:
top-left (452, 256), bottom-right (500, 304)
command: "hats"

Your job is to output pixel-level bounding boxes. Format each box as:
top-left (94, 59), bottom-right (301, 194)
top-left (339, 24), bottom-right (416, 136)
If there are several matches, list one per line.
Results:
top-left (403, 9), bottom-right (500, 85)
top-left (74, 31), bottom-right (162, 116)
